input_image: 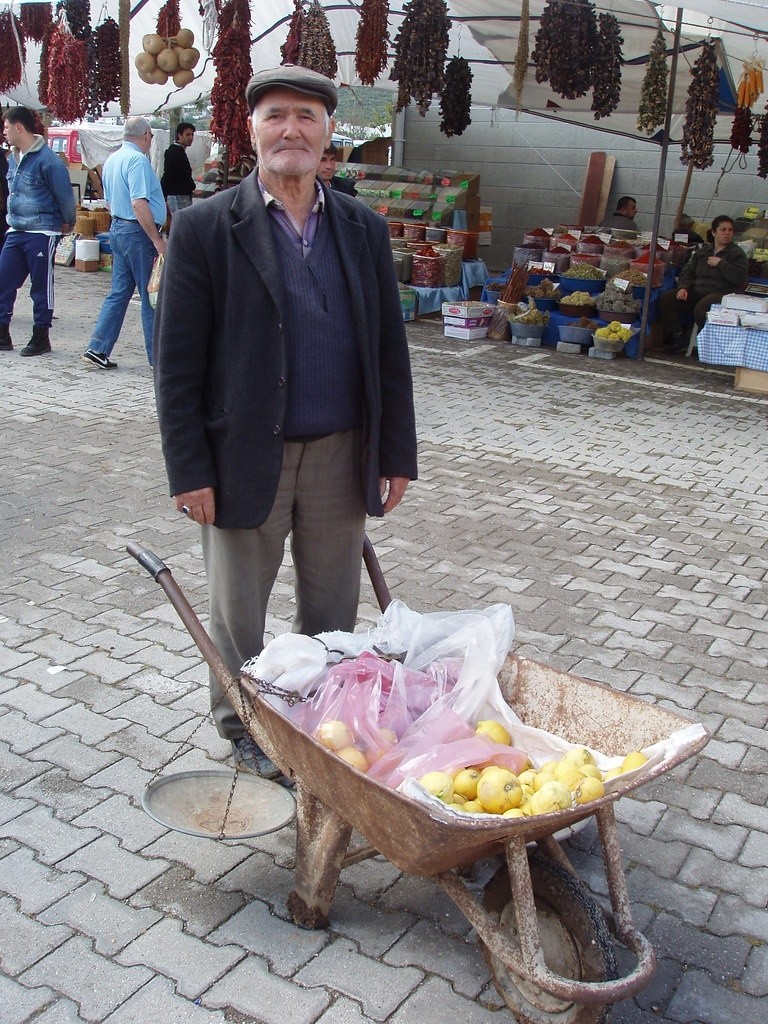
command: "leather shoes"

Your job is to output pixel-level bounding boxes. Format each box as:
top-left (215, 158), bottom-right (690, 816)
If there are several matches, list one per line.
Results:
top-left (230, 739), bottom-right (283, 779)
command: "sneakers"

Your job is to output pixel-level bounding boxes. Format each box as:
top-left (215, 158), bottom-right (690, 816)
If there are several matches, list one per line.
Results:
top-left (80, 348), bottom-right (117, 369)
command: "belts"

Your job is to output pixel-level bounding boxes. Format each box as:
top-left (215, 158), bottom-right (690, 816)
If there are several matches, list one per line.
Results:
top-left (283, 433), bottom-right (332, 442)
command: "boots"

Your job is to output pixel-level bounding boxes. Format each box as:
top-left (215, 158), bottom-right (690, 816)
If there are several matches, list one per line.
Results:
top-left (0, 325), bottom-right (13, 350)
top-left (20, 324), bottom-right (51, 355)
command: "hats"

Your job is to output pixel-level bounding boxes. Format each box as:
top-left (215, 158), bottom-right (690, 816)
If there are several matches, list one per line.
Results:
top-left (245, 64), bottom-right (338, 118)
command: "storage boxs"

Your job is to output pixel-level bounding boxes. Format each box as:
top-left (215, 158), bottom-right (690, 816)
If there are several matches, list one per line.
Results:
top-left (334, 137), bottom-right (497, 341)
top-left (54, 232), bottom-right (114, 273)
top-left (731, 206), bottom-right (768, 281)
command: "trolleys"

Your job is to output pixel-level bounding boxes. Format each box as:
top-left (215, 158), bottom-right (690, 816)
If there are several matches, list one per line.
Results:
top-left (122, 539), bottom-right (711, 1023)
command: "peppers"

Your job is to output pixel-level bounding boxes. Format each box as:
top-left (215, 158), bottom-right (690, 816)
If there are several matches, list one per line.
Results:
top-left (411, 244), bottom-right (444, 288)
top-left (156, 0), bottom-right (182, 40)
top-left (730, 107), bottom-right (754, 154)
top-left (354, 0), bottom-right (394, 87)
top-left (200, 0), bottom-right (303, 169)
top-left (0, 0), bottom-right (124, 126)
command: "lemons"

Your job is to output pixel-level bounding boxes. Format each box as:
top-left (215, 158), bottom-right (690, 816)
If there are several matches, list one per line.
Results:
top-left (317, 717), bottom-right (645, 817)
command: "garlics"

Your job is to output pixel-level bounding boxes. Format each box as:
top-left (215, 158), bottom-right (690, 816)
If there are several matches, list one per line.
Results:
top-left (135, 29), bottom-right (200, 88)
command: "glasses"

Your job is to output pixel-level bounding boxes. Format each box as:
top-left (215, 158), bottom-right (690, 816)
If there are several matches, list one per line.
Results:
top-left (144, 131), bottom-right (154, 138)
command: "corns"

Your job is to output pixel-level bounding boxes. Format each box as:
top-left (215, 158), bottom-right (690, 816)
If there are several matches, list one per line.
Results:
top-left (736, 70), bottom-right (763, 108)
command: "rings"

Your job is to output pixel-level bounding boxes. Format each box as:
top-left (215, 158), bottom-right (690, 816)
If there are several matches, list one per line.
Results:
top-left (708, 263), bottom-right (710, 266)
top-left (183, 506), bottom-right (192, 514)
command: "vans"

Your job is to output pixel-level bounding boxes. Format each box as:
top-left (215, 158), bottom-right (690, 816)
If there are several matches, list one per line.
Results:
top-left (330, 132), bottom-right (354, 147)
top-left (48, 126), bottom-right (83, 164)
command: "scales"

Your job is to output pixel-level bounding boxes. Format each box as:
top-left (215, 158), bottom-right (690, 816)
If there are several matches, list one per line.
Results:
top-left (745, 277), bottom-right (768, 295)
top-left (133, 613), bottom-right (407, 841)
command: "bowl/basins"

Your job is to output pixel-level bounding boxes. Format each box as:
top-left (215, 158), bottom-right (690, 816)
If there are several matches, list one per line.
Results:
top-left (595, 308), bottom-right (640, 324)
top-left (522, 296), bottom-right (560, 311)
top-left (485, 289), bottom-right (500, 305)
top-left (96, 234), bottom-right (112, 253)
top-left (558, 326), bottom-right (594, 344)
top-left (527, 274), bottom-right (556, 286)
top-left (510, 323), bottom-right (545, 338)
top-left (558, 273), bottom-right (606, 291)
top-left (632, 285), bottom-right (646, 299)
top-left (591, 332), bottom-right (624, 352)
top-left (556, 299), bottom-right (595, 318)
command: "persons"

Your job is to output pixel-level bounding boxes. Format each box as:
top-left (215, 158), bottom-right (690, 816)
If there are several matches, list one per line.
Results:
top-left (317, 142), bottom-right (357, 200)
top-left (657, 214), bottom-right (748, 356)
top-left (597, 196), bottom-right (640, 233)
top-left (154, 64), bottom-right (419, 780)
top-left (0, 105), bottom-right (77, 357)
top-left (160, 123), bottom-right (195, 216)
top-left (82, 117), bottom-right (167, 369)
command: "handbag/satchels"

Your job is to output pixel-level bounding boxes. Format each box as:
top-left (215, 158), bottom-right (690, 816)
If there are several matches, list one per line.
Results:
top-left (147, 253), bottom-right (165, 309)
